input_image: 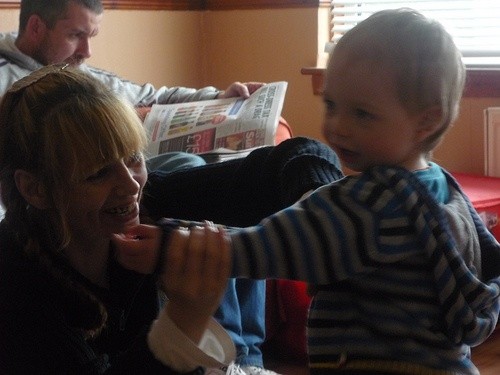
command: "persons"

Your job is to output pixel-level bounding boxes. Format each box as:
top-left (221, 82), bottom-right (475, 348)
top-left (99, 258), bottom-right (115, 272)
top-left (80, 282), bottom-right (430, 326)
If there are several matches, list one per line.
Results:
top-left (0, 63), bottom-right (236, 375)
top-left (0, 0), bottom-right (279, 375)
top-left (110, 5), bottom-right (500, 375)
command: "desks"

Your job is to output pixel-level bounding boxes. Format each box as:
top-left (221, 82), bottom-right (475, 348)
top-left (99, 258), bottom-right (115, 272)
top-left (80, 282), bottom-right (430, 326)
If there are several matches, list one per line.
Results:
top-left (273, 171), bottom-right (500, 361)
